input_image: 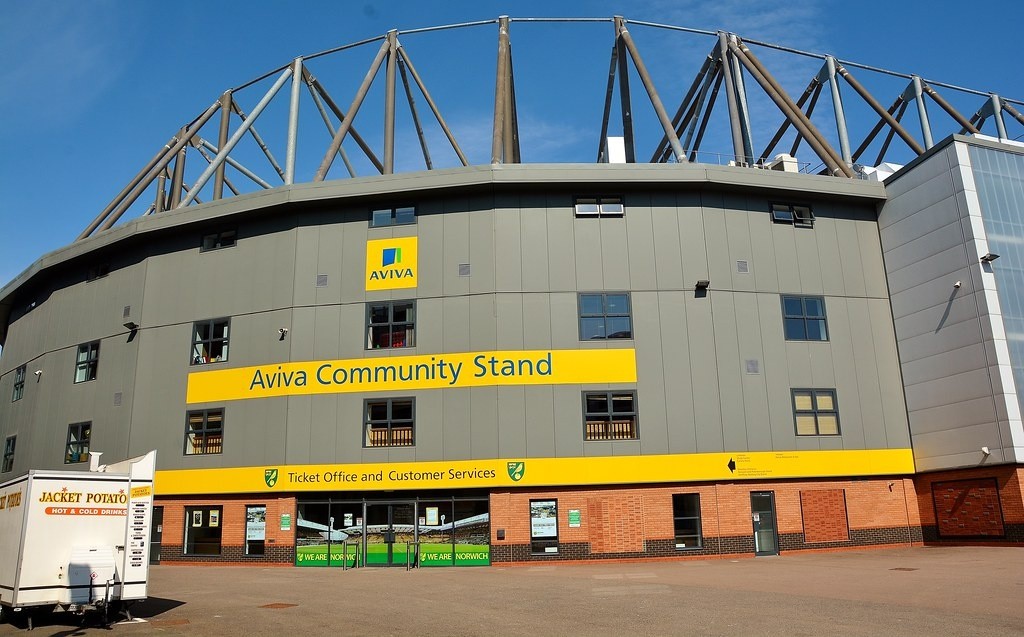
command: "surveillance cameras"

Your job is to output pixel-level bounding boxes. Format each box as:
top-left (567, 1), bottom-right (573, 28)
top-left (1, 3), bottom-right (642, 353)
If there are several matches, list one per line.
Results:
top-left (954, 281), bottom-right (961, 288)
top-left (279, 328), bottom-right (286, 333)
top-left (35, 370), bottom-right (40, 375)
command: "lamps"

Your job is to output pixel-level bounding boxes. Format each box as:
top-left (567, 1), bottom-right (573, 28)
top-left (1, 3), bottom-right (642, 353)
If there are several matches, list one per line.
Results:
top-left (123, 322), bottom-right (139, 330)
top-left (695, 280), bottom-right (710, 289)
top-left (980, 253), bottom-right (1000, 263)
